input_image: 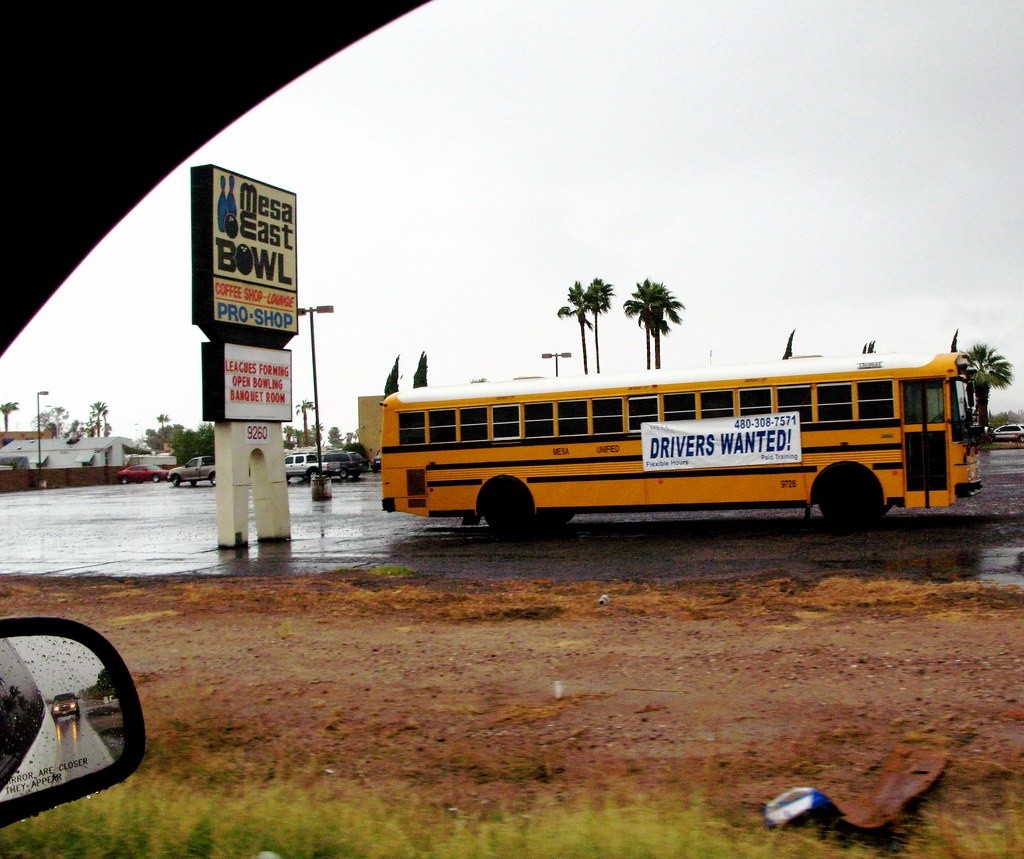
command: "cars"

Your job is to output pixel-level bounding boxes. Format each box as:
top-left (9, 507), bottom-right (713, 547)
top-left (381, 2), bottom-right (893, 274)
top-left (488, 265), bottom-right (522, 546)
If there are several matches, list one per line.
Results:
top-left (991, 423), bottom-right (1024, 443)
top-left (370, 454), bottom-right (381, 473)
top-left (114, 463), bottom-right (169, 485)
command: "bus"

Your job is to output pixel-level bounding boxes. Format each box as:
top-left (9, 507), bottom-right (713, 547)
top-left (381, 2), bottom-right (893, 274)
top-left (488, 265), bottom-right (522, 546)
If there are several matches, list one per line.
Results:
top-left (379, 351), bottom-right (989, 542)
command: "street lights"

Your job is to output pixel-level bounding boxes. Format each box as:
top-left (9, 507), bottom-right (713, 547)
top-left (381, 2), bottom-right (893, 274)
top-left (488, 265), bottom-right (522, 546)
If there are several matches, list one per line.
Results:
top-left (297, 305), bottom-right (334, 501)
top-left (542, 352), bottom-right (571, 377)
top-left (37, 391), bottom-right (49, 480)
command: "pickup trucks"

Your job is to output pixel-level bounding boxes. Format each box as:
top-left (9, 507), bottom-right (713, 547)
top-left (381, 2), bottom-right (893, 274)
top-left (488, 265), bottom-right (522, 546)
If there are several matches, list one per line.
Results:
top-left (284, 453), bottom-right (342, 483)
top-left (168, 455), bottom-right (216, 487)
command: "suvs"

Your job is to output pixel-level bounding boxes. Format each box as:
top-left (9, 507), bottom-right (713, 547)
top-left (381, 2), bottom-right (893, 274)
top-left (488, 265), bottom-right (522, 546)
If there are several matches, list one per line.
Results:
top-left (321, 451), bottom-right (368, 480)
top-left (49, 692), bottom-right (80, 722)
